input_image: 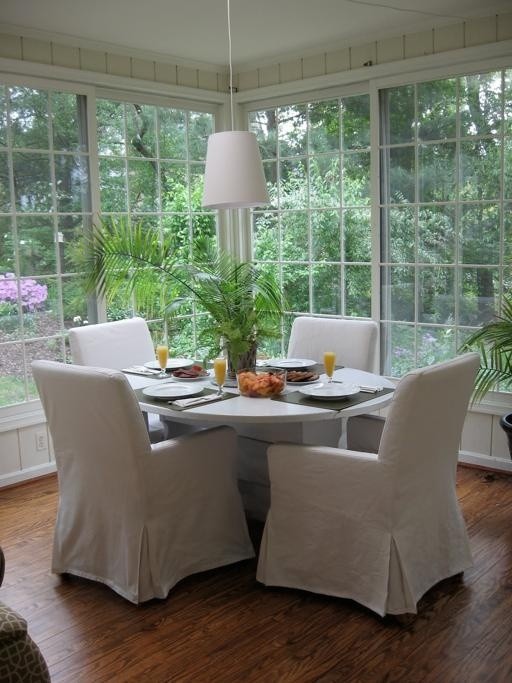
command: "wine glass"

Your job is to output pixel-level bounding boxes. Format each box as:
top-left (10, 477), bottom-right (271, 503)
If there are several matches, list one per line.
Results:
top-left (214, 358), bottom-right (226, 396)
top-left (157, 344), bottom-right (168, 377)
top-left (324, 352), bottom-right (336, 382)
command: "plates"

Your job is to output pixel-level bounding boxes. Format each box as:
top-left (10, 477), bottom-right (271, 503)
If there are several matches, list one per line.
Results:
top-left (142, 382), bottom-right (204, 397)
top-left (172, 375), bottom-right (211, 379)
top-left (144, 358), bottom-right (194, 369)
top-left (288, 372), bottom-right (320, 385)
top-left (298, 383), bottom-right (361, 400)
top-left (269, 358), bottom-right (316, 369)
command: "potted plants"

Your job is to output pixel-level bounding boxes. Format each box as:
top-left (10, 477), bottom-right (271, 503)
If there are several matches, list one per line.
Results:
top-left (74, 215), bottom-right (286, 378)
top-left (459, 289), bottom-right (512, 464)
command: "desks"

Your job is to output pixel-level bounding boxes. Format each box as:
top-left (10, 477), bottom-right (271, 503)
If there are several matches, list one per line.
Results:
top-left (110, 357), bottom-right (399, 470)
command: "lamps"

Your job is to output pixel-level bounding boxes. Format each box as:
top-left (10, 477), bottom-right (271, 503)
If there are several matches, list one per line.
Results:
top-left (200, 1), bottom-right (272, 210)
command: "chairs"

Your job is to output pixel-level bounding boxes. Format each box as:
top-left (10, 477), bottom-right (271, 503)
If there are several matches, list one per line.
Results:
top-left (279, 315), bottom-right (383, 457)
top-left (67, 318), bottom-right (187, 443)
top-left (30, 357), bottom-right (257, 612)
top-left (252, 350), bottom-right (482, 621)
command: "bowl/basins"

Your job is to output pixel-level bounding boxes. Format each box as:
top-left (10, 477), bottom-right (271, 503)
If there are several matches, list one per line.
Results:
top-left (237, 367), bottom-right (287, 396)
